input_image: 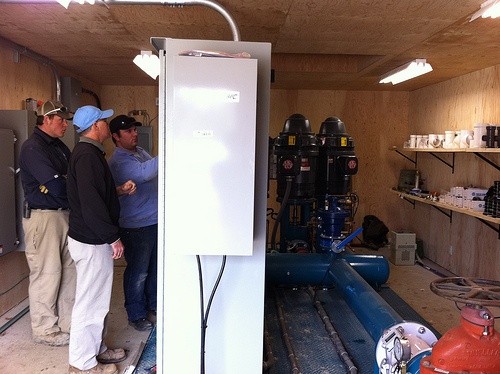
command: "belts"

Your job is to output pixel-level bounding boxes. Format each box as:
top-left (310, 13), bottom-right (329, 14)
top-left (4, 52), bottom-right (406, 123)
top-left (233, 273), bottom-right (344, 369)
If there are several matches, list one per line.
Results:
top-left (29, 205), bottom-right (70, 211)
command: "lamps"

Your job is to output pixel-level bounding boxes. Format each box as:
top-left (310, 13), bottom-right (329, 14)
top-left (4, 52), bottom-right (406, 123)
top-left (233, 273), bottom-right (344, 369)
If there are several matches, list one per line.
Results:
top-left (133, 49), bottom-right (159, 81)
top-left (379, 58), bottom-right (434, 87)
top-left (470, 0), bottom-right (500, 22)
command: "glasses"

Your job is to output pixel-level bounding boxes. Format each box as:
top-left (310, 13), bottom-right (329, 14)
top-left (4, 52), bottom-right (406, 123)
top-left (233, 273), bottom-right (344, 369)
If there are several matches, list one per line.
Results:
top-left (43, 106), bottom-right (69, 116)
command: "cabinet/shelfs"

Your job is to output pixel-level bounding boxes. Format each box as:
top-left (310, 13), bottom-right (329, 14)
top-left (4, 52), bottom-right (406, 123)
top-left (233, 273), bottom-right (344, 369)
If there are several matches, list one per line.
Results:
top-left (388, 144), bottom-right (500, 239)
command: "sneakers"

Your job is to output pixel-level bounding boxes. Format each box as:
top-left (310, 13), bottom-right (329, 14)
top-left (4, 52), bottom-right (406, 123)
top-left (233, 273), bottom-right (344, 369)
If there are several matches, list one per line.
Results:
top-left (128, 316), bottom-right (153, 331)
top-left (68, 364), bottom-right (118, 374)
top-left (34, 330), bottom-right (70, 346)
top-left (96, 348), bottom-right (126, 363)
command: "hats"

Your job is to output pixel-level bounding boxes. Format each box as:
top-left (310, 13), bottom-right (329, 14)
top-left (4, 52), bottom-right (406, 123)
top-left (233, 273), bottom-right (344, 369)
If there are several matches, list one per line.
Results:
top-left (35, 100), bottom-right (72, 119)
top-left (72, 105), bottom-right (114, 133)
top-left (109, 114), bottom-right (142, 131)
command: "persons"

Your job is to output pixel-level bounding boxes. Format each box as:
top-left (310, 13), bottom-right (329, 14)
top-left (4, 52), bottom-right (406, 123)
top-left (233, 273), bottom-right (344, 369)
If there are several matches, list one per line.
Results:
top-left (109, 114), bottom-right (157, 331)
top-left (67, 106), bottom-right (136, 374)
top-left (21, 101), bottom-right (70, 346)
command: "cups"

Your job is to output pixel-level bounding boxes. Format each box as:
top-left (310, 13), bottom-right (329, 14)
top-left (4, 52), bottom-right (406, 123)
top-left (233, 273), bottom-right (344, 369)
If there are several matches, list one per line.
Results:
top-left (409, 122), bottom-right (500, 149)
